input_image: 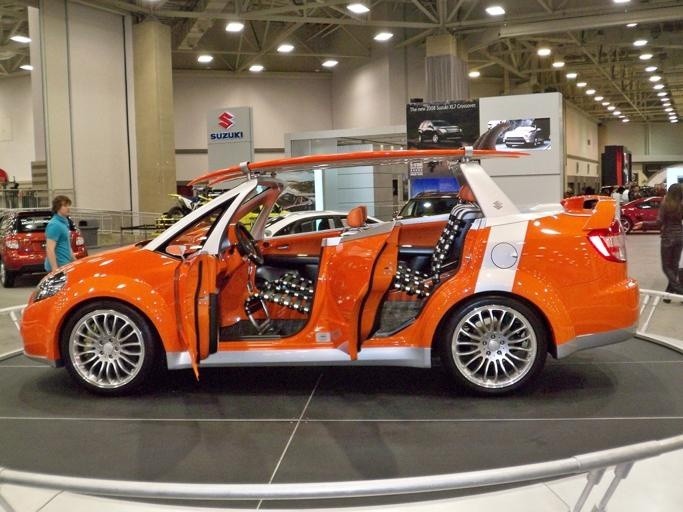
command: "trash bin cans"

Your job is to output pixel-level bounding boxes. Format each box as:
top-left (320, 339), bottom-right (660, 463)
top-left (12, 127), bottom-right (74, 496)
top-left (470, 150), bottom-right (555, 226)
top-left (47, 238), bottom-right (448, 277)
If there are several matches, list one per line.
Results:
top-left (78, 218), bottom-right (100, 249)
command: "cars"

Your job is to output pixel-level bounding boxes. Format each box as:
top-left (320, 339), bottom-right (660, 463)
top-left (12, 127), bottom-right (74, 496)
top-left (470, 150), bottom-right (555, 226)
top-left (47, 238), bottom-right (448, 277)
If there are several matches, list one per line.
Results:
top-left (600, 185), bottom-right (620, 197)
top-left (502, 125), bottom-right (547, 148)
top-left (0, 207), bottom-right (89, 287)
top-left (620, 196), bottom-right (663, 235)
top-left (19, 146), bottom-right (641, 394)
top-left (275, 191), bottom-right (313, 210)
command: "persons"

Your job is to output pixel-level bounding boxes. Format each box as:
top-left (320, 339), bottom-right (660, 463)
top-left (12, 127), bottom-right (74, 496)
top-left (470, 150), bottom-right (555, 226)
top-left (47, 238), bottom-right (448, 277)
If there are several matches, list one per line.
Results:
top-left (39, 195), bottom-right (79, 274)
top-left (565, 179), bottom-right (663, 208)
top-left (655, 183), bottom-right (683, 304)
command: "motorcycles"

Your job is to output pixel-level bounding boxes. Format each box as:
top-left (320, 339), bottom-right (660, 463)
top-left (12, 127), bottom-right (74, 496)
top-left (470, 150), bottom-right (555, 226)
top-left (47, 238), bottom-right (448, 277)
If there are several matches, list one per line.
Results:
top-left (165, 185), bottom-right (211, 218)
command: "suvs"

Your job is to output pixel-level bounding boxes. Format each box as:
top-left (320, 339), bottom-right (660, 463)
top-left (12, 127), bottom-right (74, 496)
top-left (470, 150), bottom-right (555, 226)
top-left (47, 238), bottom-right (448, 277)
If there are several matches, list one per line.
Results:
top-left (417, 120), bottom-right (465, 144)
top-left (209, 197), bottom-right (292, 232)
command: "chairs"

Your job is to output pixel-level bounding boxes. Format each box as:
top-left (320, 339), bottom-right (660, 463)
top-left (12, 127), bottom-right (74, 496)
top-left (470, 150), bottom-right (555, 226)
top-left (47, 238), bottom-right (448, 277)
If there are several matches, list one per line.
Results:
top-left (238, 203), bottom-right (366, 336)
top-left (387, 184), bottom-right (480, 300)
top-left (394, 183), bottom-right (471, 282)
top-left (269, 206), bottom-right (368, 296)
